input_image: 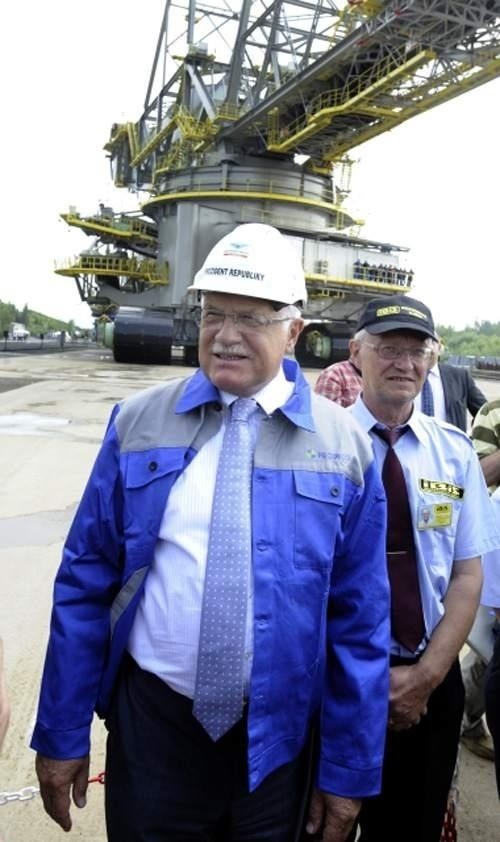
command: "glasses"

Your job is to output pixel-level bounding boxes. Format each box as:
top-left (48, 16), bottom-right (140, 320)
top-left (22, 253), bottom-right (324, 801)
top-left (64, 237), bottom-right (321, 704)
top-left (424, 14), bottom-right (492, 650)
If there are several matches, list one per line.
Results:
top-left (187, 306), bottom-right (302, 330)
top-left (357, 342), bottom-right (434, 360)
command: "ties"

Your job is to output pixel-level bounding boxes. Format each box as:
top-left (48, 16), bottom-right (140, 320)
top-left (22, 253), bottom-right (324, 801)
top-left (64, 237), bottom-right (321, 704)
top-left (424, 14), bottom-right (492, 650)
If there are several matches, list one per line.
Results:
top-left (371, 426), bottom-right (425, 654)
top-left (421, 370), bottom-right (434, 416)
top-left (192, 396), bottom-right (259, 743)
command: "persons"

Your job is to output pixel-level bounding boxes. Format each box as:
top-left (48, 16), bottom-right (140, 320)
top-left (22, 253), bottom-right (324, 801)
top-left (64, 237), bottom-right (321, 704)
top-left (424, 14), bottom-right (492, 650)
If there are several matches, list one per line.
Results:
top-left (314, 351), bottom-right (363, 408)
top-left (413, 330), bottom-right (487, 433)
top-left (345, 295), bottom-right (500, 842)
top-left (29, 223), bottom-right (392, 842)
top-left (461, 400), bottom-right (500, 761)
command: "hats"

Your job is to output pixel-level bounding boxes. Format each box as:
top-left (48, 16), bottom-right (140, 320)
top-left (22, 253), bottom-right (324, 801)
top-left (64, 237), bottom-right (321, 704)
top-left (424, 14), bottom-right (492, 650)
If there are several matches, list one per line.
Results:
top-left (355, 295), bottom-right (439, 342)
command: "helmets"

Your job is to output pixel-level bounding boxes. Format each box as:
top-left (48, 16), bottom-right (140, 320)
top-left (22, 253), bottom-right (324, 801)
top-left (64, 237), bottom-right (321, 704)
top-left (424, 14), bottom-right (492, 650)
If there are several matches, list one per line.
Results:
top-left (186, 223), bottom-right (308, 308)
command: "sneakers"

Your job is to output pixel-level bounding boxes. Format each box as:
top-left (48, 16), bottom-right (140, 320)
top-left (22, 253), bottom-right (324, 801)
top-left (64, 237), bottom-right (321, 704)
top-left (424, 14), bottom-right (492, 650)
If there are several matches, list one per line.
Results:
top-left (459, 717), bottom-right (496, 761)
